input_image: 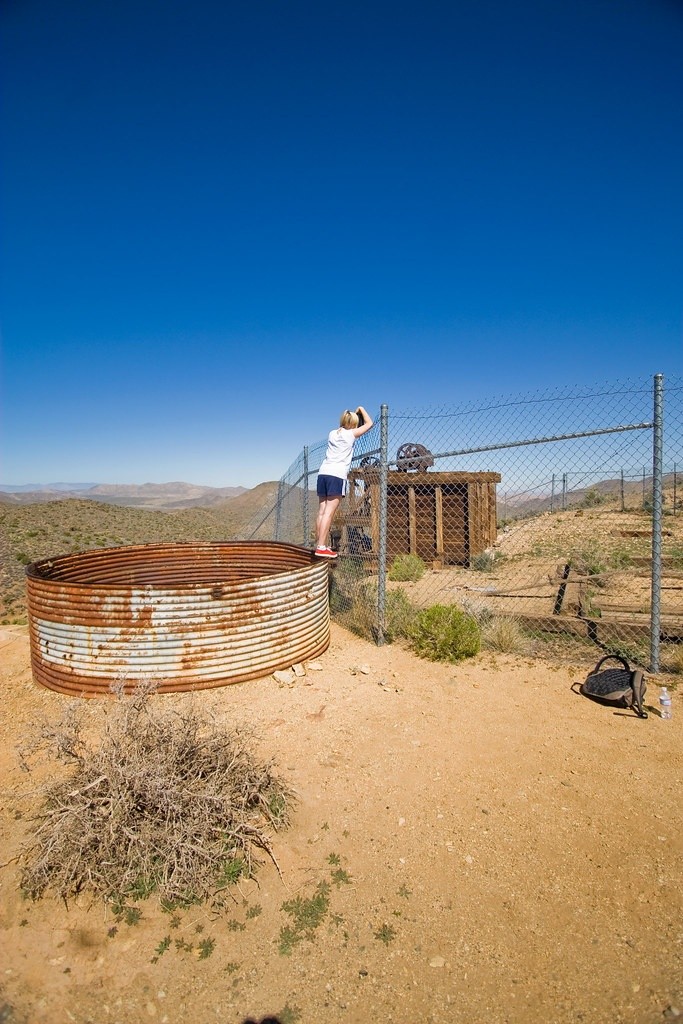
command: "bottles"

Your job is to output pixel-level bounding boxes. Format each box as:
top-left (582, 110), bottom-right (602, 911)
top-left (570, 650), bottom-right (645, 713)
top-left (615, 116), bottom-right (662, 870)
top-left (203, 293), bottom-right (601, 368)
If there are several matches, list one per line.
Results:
top-left (659, 687), bottom-right (672, 720)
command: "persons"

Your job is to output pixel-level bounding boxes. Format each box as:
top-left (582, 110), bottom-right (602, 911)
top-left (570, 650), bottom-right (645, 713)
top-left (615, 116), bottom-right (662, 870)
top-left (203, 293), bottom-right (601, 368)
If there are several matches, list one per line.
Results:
top-left (314, 406), bottom-right (373, 558)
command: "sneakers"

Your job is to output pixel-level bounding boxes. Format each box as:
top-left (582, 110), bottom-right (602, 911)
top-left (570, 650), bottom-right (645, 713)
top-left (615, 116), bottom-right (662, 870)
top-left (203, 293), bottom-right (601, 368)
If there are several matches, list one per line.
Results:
top-left (314, 548), bottom-right (338, 558)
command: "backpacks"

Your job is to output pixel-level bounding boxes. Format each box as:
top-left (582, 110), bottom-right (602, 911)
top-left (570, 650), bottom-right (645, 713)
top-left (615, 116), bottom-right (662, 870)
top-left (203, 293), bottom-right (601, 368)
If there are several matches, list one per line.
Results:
top-left (571, 654), bottom-right (648, 719)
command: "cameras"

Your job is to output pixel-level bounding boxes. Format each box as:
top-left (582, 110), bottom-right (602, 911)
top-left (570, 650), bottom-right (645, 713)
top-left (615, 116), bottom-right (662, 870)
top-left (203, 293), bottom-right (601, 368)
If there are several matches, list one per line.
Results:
top-left (357, 413), bottom-right (364, 428)
